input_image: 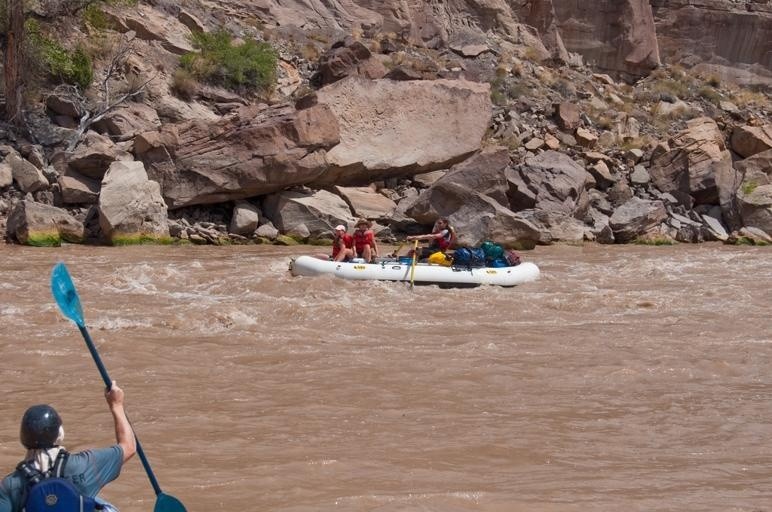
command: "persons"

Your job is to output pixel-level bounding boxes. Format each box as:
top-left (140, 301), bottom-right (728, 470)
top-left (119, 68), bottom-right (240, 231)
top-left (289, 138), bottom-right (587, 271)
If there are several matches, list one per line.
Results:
top-left (406, 216), bottom-right (455, 260)
top-left (0, 378), bottom-right (137, 512)
top-left (333, 219), bottom-right (380, 263)
top-left (332, 224), bottom-right (353, 262)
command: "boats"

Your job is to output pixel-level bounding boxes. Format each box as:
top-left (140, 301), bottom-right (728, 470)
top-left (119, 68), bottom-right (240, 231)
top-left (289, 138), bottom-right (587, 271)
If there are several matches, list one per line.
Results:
top-left (289, 255), bottom-right (541, 287)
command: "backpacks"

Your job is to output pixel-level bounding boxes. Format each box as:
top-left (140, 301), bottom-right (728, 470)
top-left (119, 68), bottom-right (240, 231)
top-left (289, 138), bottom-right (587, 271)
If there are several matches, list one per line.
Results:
top-left (16, 448), bottom-right (97, 512)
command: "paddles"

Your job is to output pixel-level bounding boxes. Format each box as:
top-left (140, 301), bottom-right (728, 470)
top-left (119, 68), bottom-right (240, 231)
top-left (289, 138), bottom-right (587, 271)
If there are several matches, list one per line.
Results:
top-left (51, 263), bottom-right (184, 512)
top-left (410, 240), bottom-right (419, 291)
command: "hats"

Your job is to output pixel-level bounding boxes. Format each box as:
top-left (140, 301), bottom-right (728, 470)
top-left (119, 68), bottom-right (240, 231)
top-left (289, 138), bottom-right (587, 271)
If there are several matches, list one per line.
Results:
top-left (354, 218), bottom-right (373, 228)
top-left (333, 224), bottom-right (346, 232)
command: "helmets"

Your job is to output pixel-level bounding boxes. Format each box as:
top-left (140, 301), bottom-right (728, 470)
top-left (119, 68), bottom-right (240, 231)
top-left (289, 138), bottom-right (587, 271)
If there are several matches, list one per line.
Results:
top-left (19, 404), bottom-right (63, 450)
